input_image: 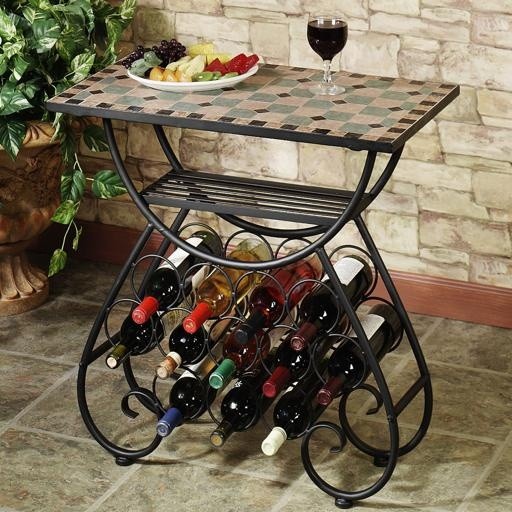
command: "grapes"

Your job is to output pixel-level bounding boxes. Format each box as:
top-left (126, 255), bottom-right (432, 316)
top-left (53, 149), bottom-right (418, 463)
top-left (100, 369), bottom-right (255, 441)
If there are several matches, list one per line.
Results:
top-left (121, 38), bottom-right (187, 77)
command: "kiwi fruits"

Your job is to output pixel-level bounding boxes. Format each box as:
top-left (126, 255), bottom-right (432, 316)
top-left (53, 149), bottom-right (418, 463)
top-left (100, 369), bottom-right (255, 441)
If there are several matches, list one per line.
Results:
top-left (194, 70), bottom-right (239, 81)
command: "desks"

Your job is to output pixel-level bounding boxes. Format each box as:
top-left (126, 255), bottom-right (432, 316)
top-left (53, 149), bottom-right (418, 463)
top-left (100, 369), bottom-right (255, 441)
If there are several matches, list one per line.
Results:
top-left (45, 46), bottom-right (461, 509)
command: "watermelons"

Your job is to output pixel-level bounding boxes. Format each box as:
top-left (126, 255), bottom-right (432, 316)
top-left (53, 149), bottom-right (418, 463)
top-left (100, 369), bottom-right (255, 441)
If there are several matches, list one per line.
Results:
top-left (202, 53), bottom-right (260, 74)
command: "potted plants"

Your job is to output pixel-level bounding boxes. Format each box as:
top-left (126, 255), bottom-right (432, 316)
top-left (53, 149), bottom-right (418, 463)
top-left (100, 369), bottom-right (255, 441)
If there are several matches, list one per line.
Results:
top-left (0, 0), bottom-right (137, 317)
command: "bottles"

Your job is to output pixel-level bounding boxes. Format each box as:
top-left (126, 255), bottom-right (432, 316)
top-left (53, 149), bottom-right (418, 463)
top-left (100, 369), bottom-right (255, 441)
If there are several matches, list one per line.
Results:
top-left (208, 307), bottom-right (296, 390)
top-left (155, 296), bottom-right (247, 381)
top-left (262, 313), bottom-right (349, 398)
top-left (182, 237), bottom-right (271, 335)
top-left (235, 246), bottom-right (325, 343)
top-left (262, 331), bottom-right (335, 456)
top-left (131, 231), bottom-right (223, 324)
top-left (208, 348), bottom-right (291, 448)
top-left (156, 334), bottom-right (233, 437)
top-left (105, 292), bottom-right (197, 369)
top-left (291, 254), bottom-right (373, 352)
top-left (317, 304), bottom-right (402, 406)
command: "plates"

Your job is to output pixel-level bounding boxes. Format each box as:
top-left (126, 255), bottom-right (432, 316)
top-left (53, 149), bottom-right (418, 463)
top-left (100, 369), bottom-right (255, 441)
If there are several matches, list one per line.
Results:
top-left (125, 49), bottom-right (263, 91)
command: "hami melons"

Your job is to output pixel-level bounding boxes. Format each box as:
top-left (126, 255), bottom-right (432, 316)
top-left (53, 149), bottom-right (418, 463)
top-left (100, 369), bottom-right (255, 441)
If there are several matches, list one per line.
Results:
top-left (150, 54), bottom-right (205, 82)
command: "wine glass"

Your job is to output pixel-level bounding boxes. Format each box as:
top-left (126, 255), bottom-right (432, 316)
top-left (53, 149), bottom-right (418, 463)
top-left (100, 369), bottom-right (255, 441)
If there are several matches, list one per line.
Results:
top-left (306, 11), bottom-right (349, 95)
